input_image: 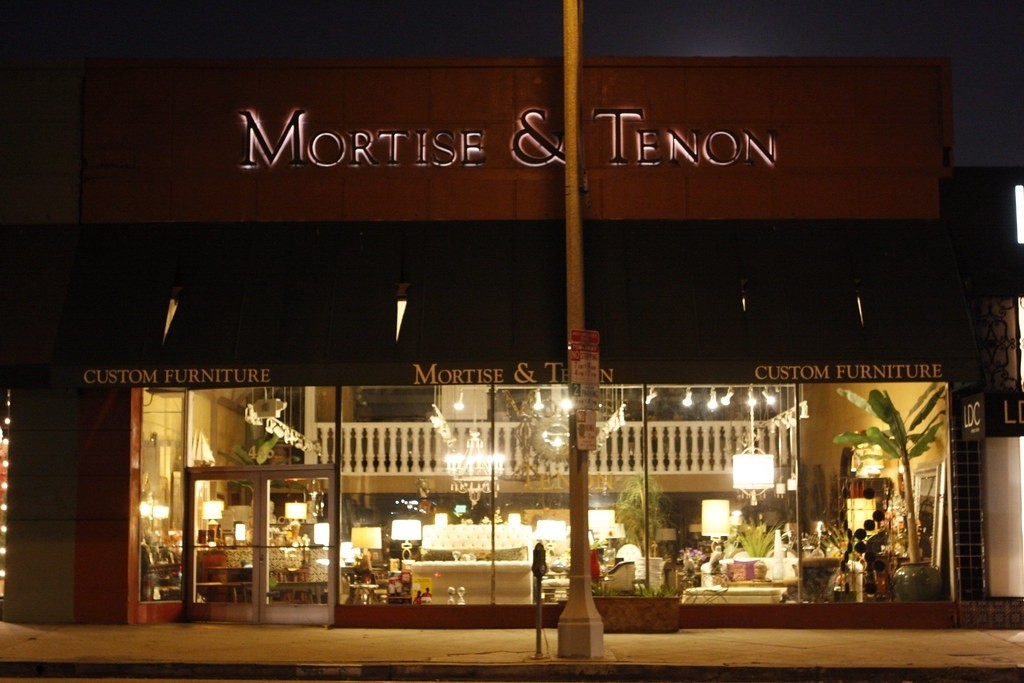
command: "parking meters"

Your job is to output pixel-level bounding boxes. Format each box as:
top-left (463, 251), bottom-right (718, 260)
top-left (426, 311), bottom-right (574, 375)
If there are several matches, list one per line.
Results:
top-left (531, 544), bottom-right (549, 659)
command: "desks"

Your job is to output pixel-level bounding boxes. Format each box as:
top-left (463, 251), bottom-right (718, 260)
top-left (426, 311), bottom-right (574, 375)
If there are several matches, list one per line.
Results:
top-left (206, 567), bottom-right (253, 603)
top-left (202, 548), bottom-right (329, 602)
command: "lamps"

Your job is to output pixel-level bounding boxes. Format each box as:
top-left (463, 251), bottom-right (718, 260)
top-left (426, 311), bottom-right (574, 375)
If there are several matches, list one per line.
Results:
top-left (776, 385), bottom-right (797, 499)
top-left (536, 519), bottom-right (567, 572)
top-left (202, 500), bottom-right (223, 541)
top-left (733, 388), bottom-right (774, 506)
top-left (655, 529), bottom-right (676, 562)
top-left (351, 528), bottom-right (382, 571)
top-left (771, 400), bottom-right (810, 434)
top-left (689, 525), bottom-right (702, 539)
top-left (605, 524), bottom-right (626, 548)
top-left (285, 500), bottom-right (307, 547)
top-left (391, 519), bottom-right (421, 559)
top-left (702, 500), bottom-right (730, 574)
top-left (140, 494), bottom-right (170, 545)
top-left (588, 510), bottom-right (615, 548)
top-left (367, 535), bottom-right (368, 536)
top-left (851, 442), bottom-right (884, 478)
top-left (442, 389), bottom-right (506, 510)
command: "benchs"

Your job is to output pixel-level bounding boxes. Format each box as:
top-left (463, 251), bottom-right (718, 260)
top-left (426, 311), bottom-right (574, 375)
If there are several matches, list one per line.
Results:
top-left (683, 586), bottom-right (781, 604)
top-left (193, 581), bottom-right (328, 604)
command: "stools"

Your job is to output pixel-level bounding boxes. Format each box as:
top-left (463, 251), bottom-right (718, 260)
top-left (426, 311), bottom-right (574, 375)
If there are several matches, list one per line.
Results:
top-left (274, 568), bottom-right (308, 602)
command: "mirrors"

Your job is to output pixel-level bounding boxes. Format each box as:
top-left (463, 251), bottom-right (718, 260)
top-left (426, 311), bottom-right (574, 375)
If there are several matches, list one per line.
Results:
top-left (914, 465), bottom-right (941, 568)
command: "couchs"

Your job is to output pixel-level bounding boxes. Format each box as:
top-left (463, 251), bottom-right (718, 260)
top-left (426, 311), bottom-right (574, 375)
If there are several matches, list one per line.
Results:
top-left (412, 524), bottom-right (534, 604)
top-left (700, 559), bottom-right (798, 587)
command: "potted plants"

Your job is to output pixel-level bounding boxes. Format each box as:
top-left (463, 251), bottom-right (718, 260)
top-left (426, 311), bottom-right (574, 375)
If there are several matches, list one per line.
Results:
top-left (590, 584), bottom-right (682, 633)
top-left (730, 517), bottom-right (794, 581)
top-left (614, 470), bottom-right (681, 596)
top-left (833, 381), bottom-right (947, 601)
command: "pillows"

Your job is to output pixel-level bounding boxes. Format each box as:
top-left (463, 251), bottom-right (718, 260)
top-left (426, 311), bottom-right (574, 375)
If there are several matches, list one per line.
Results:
top-left (725, 560), bottom-right (757, 582)
top-left (424, 546), bottom-right (528, 561)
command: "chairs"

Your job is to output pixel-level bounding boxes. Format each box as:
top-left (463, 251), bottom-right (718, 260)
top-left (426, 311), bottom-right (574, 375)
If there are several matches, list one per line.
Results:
top-left (607, 561), bottom-right (635, 591)
top-left (680, 573), bottom-right (729, 605)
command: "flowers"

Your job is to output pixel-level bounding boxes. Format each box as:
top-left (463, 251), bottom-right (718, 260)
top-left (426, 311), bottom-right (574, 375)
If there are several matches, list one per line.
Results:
top-left (677, 547), bottom-right (706, 568)
